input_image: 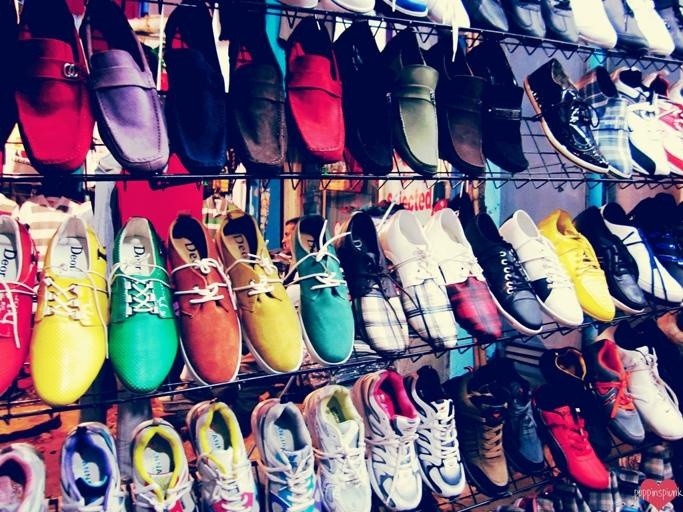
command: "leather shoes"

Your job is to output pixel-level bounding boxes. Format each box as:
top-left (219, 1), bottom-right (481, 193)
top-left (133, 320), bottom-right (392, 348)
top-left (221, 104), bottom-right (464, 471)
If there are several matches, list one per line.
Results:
top-left (108, 213), bottom-right (178, 396)
top-left (523, 58), bottom-right (608, 175)
top-left (28, 215), bottom-right (112, 408)
top-left (0, 213), bottom-right (39, 402)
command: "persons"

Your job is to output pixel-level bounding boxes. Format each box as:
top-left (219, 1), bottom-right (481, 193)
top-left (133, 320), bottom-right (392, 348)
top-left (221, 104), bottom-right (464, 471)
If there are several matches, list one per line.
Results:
top-left (281, 217), bottom-right (302, 254)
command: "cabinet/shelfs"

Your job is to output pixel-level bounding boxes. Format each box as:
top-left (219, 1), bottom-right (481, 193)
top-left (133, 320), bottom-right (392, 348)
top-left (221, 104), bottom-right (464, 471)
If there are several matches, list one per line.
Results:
top-left (0, 0), bottom-right (683, 512)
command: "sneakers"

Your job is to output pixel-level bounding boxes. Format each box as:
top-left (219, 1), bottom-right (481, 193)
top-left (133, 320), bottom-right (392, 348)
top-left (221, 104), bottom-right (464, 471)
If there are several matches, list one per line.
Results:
top-left (482, 356), bottom-right (546, 476)
top-left (582, 339), bottom-right (644, 447)
top-left (446, 375), bottom-right (511, 498)
top-left (186, 399), bottom-right (263, 511)
top-left (531, 383), bottom-right (609, 492)
top-left (424, 194), bottom-right (681, 335)
top-left (0, 442), bottom-right (49, 511)
top-left (57, 422), bottom-right (129, 512)
top-left (577, 65), bottom-right (683, 180)
top-left (280, 0), bottom-right (680, 58)
top-left (352, 369), bottom-right (425, 512)
top-left (593, 320), bottom-right (683, 442)
top-left (303, 383), bottom-right (374, 512)
top-left (538, 346), bottom-right (611, 460)
top-left (14, 0), bottom-right (93, 174)
top-left (129, 413), bottom-right (199, 511)
top-left (169, 206), bottom-right (458, 386)
top-left (403, 365), bottom-right (466, 499)
top-left (249, 396), bottom-right (323, 512)
